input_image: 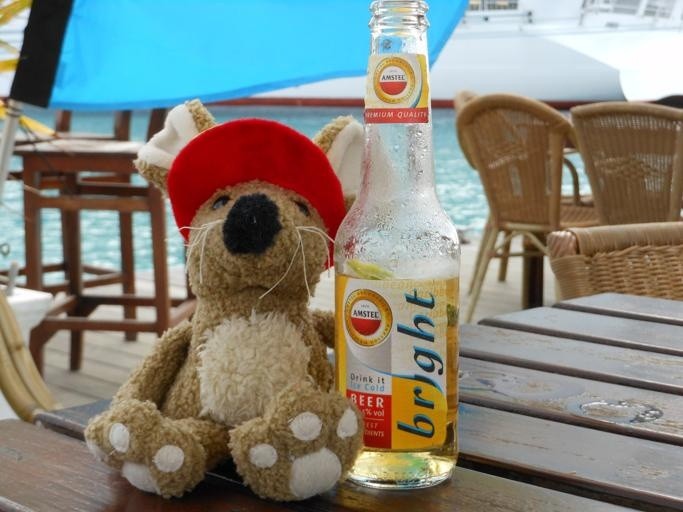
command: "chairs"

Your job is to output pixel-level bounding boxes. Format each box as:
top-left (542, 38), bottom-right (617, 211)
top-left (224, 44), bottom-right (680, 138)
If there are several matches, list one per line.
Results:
top-left (452, 90), bottom-right (681, 329)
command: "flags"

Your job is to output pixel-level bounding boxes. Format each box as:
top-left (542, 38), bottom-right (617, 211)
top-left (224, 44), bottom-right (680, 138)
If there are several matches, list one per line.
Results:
top-left (10, 0), bottom-right (473, 113)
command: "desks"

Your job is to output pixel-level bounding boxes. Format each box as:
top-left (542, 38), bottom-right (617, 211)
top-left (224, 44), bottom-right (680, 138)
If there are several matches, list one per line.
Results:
top-left (0, 289), bottom-right (683, 512)
top-left (12, 138), bottom-right (196, 384)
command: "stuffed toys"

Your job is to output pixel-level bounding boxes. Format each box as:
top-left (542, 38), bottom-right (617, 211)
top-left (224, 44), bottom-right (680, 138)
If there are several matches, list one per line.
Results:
top-left (79, 96), bottom-right (392, 502)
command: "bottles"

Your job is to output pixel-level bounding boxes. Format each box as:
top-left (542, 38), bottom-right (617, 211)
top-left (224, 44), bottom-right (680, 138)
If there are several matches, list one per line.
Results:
top-left (334, 1), bottom-right (459, 487)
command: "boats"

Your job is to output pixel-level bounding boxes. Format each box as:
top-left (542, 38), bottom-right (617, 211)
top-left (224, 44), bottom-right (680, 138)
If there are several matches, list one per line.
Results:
top-left (218, 1), bottom-right (682, 117)
